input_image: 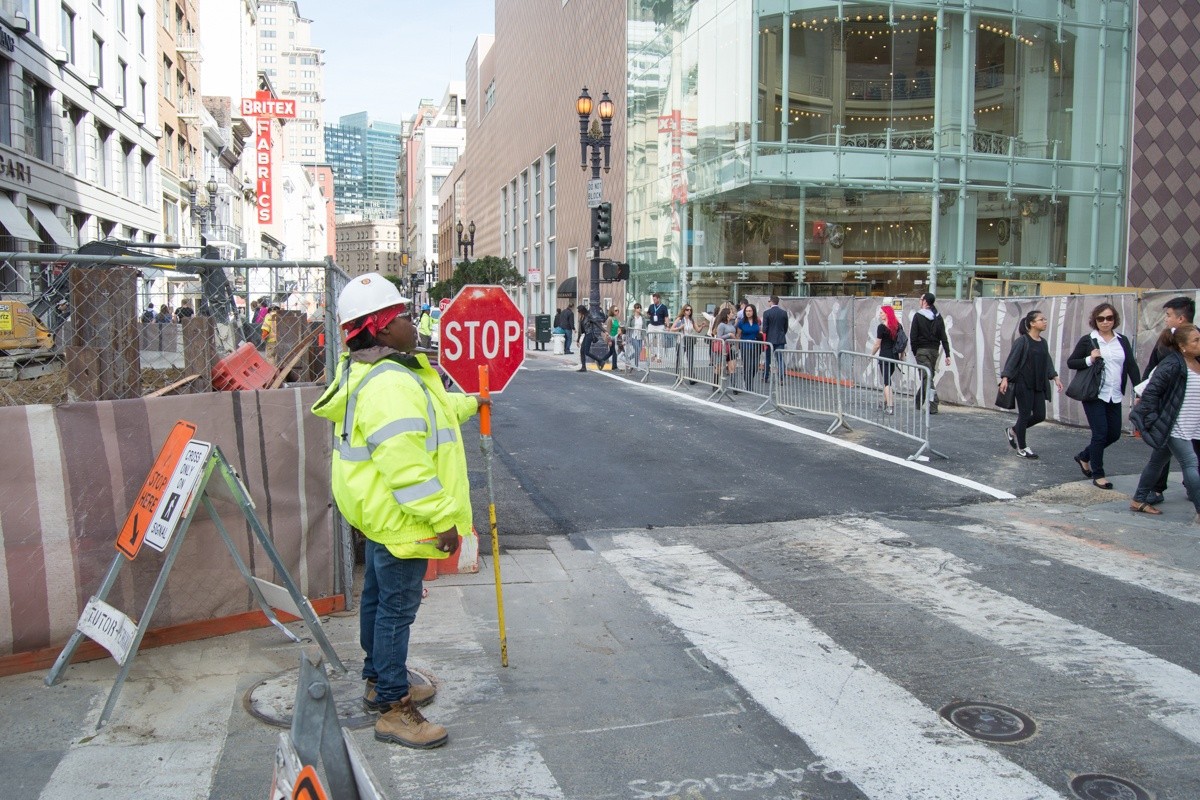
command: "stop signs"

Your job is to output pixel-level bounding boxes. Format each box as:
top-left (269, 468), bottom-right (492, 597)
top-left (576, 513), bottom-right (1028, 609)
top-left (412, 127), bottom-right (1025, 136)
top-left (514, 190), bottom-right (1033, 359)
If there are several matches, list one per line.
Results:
top-left (439, 285), bottom-right (525, 398)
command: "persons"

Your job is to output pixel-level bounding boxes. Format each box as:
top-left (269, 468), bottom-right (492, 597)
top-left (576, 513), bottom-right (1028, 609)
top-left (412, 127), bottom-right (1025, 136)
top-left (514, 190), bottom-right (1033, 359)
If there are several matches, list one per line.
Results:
top-left (1129, 324), bottom-right (1200, 517)
top-left (256, 297), bottom-right (269, 323)
top-left (626, 304), bottom-right (646, 372)
top-left (418, 304), bottom-right (440, 349)
top-left (316, 272), bottom-right (495, 751)
top-left (259, 304), bottom-right (282, 366)
top-left (560, 303), bottom-right (577, 354)
top-left (910, 293), bottom-right (951, 414)
top-left (596, 305), bottom-right (623, 371)
top-left (1135, 296), bottom-right (1200, 504)
top-left (155, 304), bottom-right (174, 323)
top-left (671, 304), bottom-right (705, 384)
top-left (250, 301), bottom-right (260, 322)
top-left (1067, 303), bottom-right (1140, 490)
top-left (866, 306), bottom-right (905, 412)
top-left (763, 295), bottom-right (789, 384)
top-left (141, 303), bottom-right (157, 323)
top-left (701, 299), bottom-right (762, 395)
top-left (174, 299), bottom-right (194, 324)
top-left (554, 308), bottom-right (562, 334)
top-left (52, 301), bottom-right (70, 338)
top-left (646, 295), bottom-right (670, 362)
top-left (1000, 310), bottom-right (1063, 459)
top-left (575, 305), bottom-right (609, 372)
top-left (856, 61), bottom-right (999, 101)
top-left (608, 327), bottom-right (634, 371)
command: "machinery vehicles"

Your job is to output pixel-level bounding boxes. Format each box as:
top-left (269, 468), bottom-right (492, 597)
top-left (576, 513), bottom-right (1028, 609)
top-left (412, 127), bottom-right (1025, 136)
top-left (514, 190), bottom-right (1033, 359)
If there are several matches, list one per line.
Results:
top-left (1, 237), bottom-right (280, 394)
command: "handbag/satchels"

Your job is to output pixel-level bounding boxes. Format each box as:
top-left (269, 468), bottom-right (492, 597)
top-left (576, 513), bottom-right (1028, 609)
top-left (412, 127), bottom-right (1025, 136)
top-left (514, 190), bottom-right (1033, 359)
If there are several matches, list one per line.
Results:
top-left (704, 335), bottom-right (711, 344)
top-left (712, 341), bottom-right (729, 352)
top-left (994, 383), bottom-right (1016, 409)
top-left (894, 323), bottom-right (908, 353)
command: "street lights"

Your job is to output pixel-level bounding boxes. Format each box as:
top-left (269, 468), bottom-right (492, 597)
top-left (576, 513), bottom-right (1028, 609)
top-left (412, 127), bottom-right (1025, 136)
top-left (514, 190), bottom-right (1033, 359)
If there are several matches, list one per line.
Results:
top-left (298, 266), bottom-right (308, 294)
top-left (575, 84), bottom-right (618, 364)
top-left (267, 241), bottom-right (284, 306)
top-left (455, 218), bottom-right (476, 265)
top-left (186, 173), bottom-right (217, 264)
top-left (423, 259), bottom-right (436, 308)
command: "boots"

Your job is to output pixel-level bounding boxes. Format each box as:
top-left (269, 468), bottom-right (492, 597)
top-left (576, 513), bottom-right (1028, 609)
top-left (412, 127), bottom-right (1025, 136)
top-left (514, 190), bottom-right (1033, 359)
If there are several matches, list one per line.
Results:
top-left (374, 695), bottom-right (448, 750)
top-left (363, 677), bottom-right (435, 709)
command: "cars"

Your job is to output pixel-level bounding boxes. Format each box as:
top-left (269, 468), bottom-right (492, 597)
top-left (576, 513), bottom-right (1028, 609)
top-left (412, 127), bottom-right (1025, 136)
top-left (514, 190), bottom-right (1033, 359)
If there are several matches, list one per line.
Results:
top-left (414, 305), bottom-right (444, 363)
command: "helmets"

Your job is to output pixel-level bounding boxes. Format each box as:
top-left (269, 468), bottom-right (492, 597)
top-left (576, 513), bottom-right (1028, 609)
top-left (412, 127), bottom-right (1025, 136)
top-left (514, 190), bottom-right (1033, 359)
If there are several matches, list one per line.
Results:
top-left (338, 272), bottom-right (410, 324)
top-left (421, 303), bottom-right (430, 310)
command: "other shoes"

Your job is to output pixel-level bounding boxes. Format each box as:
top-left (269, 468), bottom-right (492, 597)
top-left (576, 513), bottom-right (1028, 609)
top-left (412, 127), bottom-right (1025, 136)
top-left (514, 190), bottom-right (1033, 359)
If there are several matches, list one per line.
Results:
top-left (563, 350), bottom-right (662, 372)
top-left (676, 374), bottom-right (784, 395)
top-left (1074, 453), bottom-right (1092, 477)
top-left (1146, 491), bottom-right (1164, 504)
top-left (878, 392), bottom-right (940, 415)
top-left (1066, 357), bottom-right (1105, 400)
top-left (1093, 478), bottom-right (1113, 489)
top-left (1131, 501), bottom-right (1162, 513)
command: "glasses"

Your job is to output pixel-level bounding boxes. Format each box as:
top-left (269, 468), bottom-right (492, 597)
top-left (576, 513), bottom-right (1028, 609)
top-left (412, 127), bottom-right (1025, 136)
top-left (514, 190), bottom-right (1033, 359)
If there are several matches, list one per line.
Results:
top-left (636, 307), bottom-right (642, 309)
top-left (768, 300), bottom-right (771, 302)
top-left (685, 309), bottom-right (692, 311)
top-left (1034, 318), bottom-right (1048, 322)
top-left (614, 310), bottom-right (619, 312)
top-left (1096, 315), bottom-right (1114, 322)
top-left (396, 312), bottom-right (411, 320)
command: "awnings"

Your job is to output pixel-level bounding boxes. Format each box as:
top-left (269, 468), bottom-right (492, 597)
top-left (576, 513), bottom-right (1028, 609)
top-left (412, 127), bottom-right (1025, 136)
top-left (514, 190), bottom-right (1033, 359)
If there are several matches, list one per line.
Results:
top-left (558, 277), bottom-right (576, 299)
top-left (0, 192), bottom-right (44, 244)
top-left (27, 200), bottom-right (78, 250)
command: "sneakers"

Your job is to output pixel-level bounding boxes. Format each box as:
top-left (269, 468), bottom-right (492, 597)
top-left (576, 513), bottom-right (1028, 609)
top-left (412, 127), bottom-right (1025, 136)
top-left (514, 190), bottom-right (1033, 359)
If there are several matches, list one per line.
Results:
top-left (1017, 447), bottom-right (1039, 458)
top-left (1005, 427), bottom-right (1017, 449)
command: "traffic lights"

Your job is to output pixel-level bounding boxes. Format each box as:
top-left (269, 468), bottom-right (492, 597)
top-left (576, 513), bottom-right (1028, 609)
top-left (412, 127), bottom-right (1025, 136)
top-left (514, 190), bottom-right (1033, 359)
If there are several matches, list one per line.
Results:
top-left (601, 261), bottom-right (628, 281)
top-left (594, 200), bottom-right (613, 250)
